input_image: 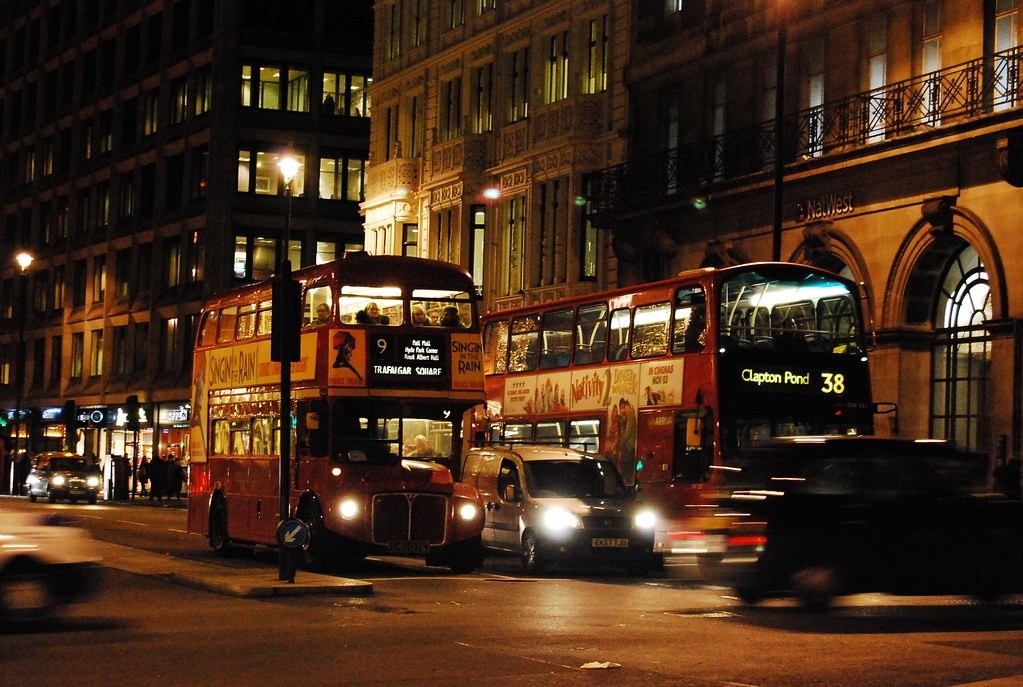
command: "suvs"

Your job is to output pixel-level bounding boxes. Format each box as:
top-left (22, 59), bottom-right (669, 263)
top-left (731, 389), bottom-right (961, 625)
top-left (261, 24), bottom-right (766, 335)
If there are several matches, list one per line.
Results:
top-left (714, 432), bottom-right (1021, 610)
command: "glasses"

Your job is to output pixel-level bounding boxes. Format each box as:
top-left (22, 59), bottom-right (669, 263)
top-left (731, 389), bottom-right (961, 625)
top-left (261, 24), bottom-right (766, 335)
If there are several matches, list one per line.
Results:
top-left (367, 307), bottom-right (378, 311)
top-left (317, 310), bottom-right (324, 313)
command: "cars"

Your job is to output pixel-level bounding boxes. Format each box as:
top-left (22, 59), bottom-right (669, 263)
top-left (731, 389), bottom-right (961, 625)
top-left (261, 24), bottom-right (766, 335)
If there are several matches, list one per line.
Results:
top-left (22, 450), bottom-right (104, 505)
top-left (1, 508), bottom-right (103, 635)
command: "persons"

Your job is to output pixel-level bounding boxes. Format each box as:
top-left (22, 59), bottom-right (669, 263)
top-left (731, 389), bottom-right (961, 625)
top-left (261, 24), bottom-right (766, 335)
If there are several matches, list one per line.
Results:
top-left (356, 303), bottom-right (390, 325)
top-left (606, 398), bottom-right (635, 471)
top-left (524, 378), bottom-right (568, 414)
top-left (118, 453), bottom-right (187, 502)
top-left (406, 434), bottom-right (445, 457)
top-left (413, 305), bottom-right (465, 328)
top-left (190, 361), bottom-right (205, 450)
top-left (333, 339), bottom-right (363, 381)
top-left (644, 386), bottom-right (674, 405)
top-left (309, 302), bottom-right (329, 327)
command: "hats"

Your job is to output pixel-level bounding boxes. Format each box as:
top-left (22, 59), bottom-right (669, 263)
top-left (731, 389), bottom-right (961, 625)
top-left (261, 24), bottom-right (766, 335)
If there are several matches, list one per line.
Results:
top-left (443, 307), bottom-right (458, 314)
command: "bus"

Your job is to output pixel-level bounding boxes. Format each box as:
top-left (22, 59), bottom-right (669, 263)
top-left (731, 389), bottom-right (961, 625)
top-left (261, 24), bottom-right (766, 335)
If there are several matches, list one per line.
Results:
top-left (189, 250), bottom-right (488, 577)
top-left (454, 259), bottom-right (904, 590)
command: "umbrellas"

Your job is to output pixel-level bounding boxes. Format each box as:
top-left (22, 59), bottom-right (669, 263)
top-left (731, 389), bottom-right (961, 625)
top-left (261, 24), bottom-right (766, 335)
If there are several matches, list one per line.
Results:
top-left (333, 330), bottom-right (355, 348)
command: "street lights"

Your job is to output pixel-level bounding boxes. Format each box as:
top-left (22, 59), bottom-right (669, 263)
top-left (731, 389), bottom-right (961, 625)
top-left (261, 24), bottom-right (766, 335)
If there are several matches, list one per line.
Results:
top-left (10, 247), bottom-right (39, 496)
top-left (267, 141), bottom-right (307, 580)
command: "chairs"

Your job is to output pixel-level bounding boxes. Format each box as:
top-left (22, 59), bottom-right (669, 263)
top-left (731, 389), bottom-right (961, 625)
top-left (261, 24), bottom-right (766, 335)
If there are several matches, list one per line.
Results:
top-left (405, 444), bottom-right (418, 456)
top-left (717, 327), bottom-right (864, 355)
top-left (346, 303), bottom-right (403, 326)
top-left (522, 341), bottom-right (632, 370)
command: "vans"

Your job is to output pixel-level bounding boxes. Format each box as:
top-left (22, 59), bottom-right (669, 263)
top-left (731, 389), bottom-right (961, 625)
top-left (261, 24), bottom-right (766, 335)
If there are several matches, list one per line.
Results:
top-left (457, 434), bottom-right (656, 579)
